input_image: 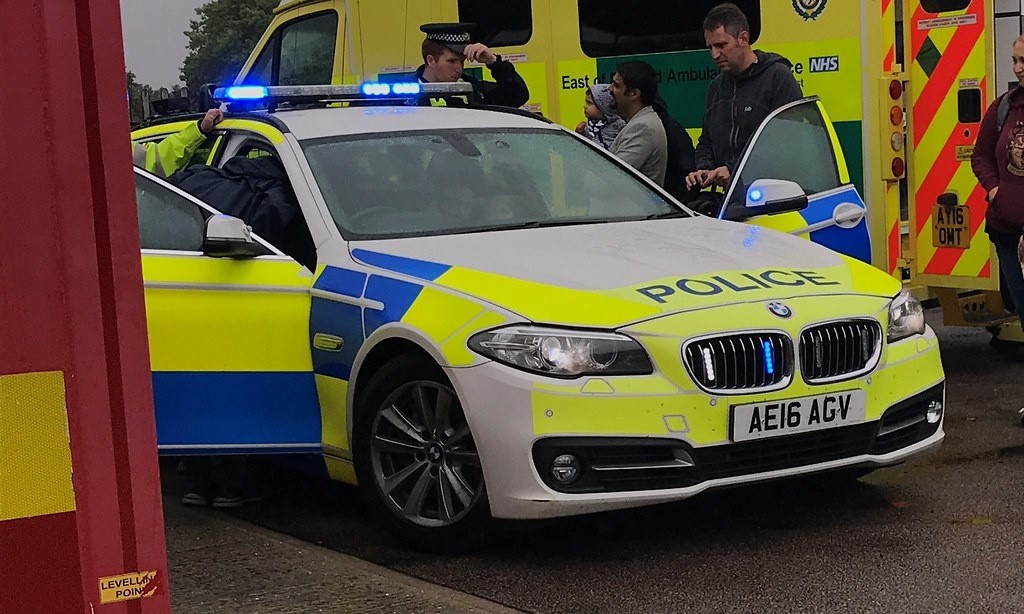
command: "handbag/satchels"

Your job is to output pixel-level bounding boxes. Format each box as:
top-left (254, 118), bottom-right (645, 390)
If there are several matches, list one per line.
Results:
top-left (680, 177), bottom-right (724, 217)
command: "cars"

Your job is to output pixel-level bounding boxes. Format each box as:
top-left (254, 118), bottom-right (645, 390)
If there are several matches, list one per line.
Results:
top-left (112, 75), bottom-right (950, 549)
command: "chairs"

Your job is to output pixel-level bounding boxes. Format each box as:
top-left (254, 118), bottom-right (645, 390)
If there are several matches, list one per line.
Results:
top-left (427, 153), bottom-right (554, 228)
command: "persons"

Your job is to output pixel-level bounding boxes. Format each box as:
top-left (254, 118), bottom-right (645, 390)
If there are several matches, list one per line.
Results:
top-left (131, 108), bottom-right (319, 508)
top-left (971, 36), bottom-right (1024, 424)
top-left (576, 3), bottom-right (804, 209)
top-left (395, 22), bottom-right (531, 171)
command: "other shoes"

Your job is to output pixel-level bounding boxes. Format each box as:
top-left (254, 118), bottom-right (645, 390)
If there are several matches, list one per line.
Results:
top-left (182, 480), bottom-right (207, 506)
top-left (213, 473), bottom-right (268, 507)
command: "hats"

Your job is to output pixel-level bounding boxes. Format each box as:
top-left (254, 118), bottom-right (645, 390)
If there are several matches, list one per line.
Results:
top-left (420, 23), bottom-right (476, 54)
top-left (589, 84), bottom-right (618, 120)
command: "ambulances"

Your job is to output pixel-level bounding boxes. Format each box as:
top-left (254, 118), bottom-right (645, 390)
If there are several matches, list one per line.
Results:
top-left (209, 0), bottom-right (1022, 326)
